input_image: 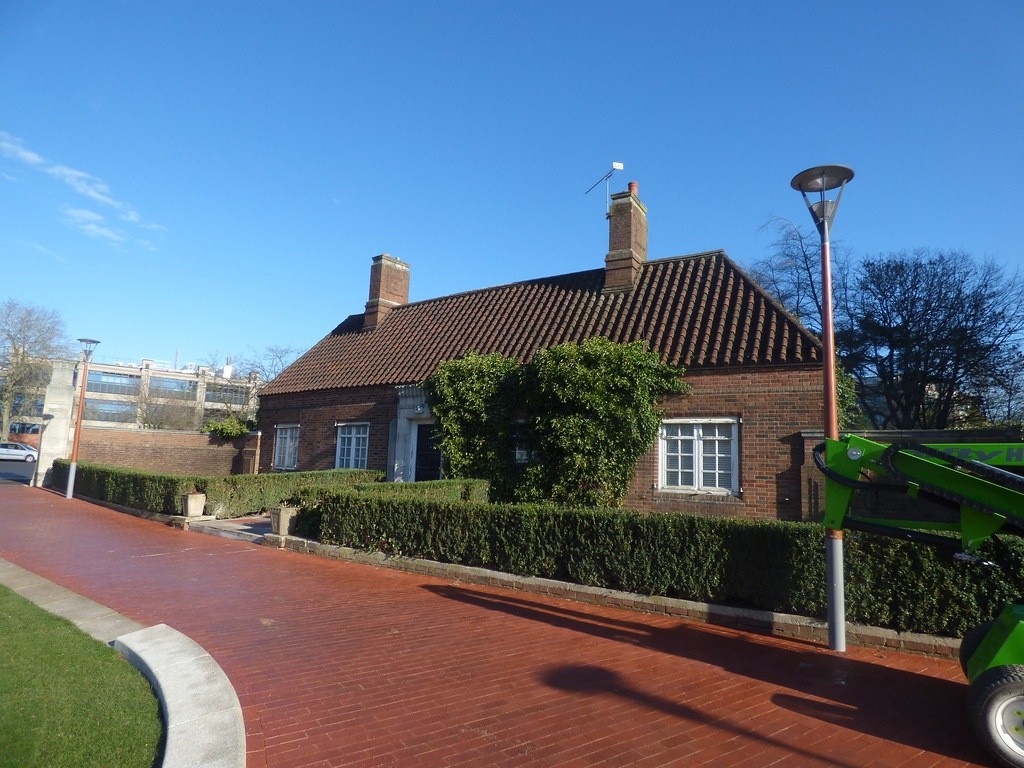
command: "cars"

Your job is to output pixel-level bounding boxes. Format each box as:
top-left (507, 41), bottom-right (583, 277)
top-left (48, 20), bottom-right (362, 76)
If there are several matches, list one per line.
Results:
top-left (0, 441), bottom-right (39, 463)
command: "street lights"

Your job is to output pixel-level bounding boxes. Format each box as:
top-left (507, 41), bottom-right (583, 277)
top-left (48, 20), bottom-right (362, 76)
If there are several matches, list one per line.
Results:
top-left (65, 338), bottom-right (102, 500)
top-left (790, 164), bottom-right (856, 653)
